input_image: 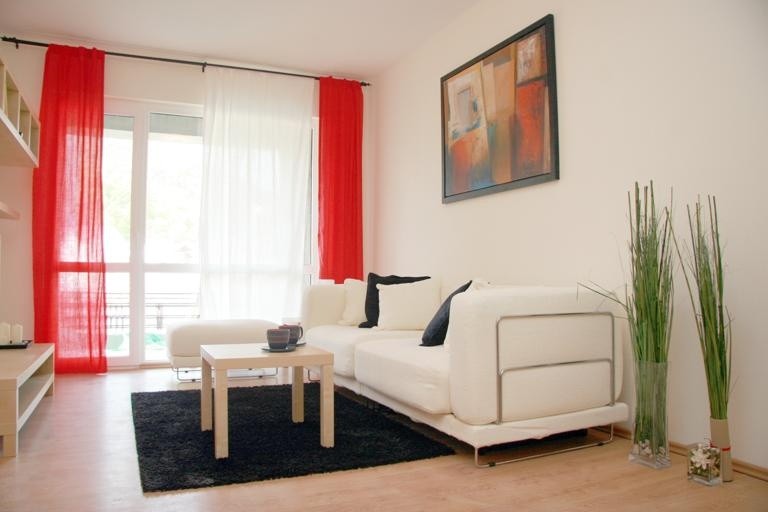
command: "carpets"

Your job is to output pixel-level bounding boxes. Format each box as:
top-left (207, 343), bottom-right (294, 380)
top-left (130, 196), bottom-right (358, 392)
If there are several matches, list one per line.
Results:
top-left (130, 382), bottom-right (462, 493)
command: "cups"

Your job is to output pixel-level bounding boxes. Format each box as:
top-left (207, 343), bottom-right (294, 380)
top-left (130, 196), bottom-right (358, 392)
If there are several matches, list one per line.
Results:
top-left (266, 328), bottom-right (291, 349)
top-left (278, 323), bottom-right (303, 344)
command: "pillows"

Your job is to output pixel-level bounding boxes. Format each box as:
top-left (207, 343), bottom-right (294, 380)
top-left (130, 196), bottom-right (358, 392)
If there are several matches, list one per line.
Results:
top-left (338, 271), bottom-right (483, 348)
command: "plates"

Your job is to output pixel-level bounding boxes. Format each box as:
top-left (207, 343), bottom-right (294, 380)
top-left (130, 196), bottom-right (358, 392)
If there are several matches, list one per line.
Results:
top-left (260, 345), bottom-right (297, 352)
top-left (286, 342), bottom-right (307, 347)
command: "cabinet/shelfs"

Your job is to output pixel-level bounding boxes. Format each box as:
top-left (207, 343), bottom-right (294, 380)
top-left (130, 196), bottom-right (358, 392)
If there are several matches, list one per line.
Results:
top-left (0, 52), bottom-right (55, 459)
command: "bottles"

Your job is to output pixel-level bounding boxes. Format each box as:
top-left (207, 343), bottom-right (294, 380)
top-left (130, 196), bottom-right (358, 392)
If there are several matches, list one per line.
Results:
top-left (11, 323), bottom-right (23, 343)
top-left (1, 323), bottom-right (10, 343)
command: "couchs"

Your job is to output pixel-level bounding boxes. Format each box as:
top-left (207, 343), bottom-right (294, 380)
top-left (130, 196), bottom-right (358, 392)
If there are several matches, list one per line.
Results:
top-left (298, 281), bottom-right (631, 469)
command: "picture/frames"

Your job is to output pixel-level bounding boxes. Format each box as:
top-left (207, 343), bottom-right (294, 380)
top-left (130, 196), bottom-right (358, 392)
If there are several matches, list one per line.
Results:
top-left (439, 12), bottom-right (559, 205)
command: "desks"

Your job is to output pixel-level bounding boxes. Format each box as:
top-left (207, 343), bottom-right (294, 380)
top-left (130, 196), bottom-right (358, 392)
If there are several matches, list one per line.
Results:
top-left (198, 343), bottom-right (337, 460)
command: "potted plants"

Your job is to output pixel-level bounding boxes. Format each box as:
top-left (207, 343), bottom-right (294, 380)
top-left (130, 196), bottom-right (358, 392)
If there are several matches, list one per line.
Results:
top-left (576, 179), bottom-right (676, 471)
top-left (664, 192), bottom-right (736, 485)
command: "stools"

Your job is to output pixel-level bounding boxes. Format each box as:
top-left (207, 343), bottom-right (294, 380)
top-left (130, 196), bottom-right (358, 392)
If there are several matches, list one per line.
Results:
top-left (167, 317), bottom-right (293, 381)
